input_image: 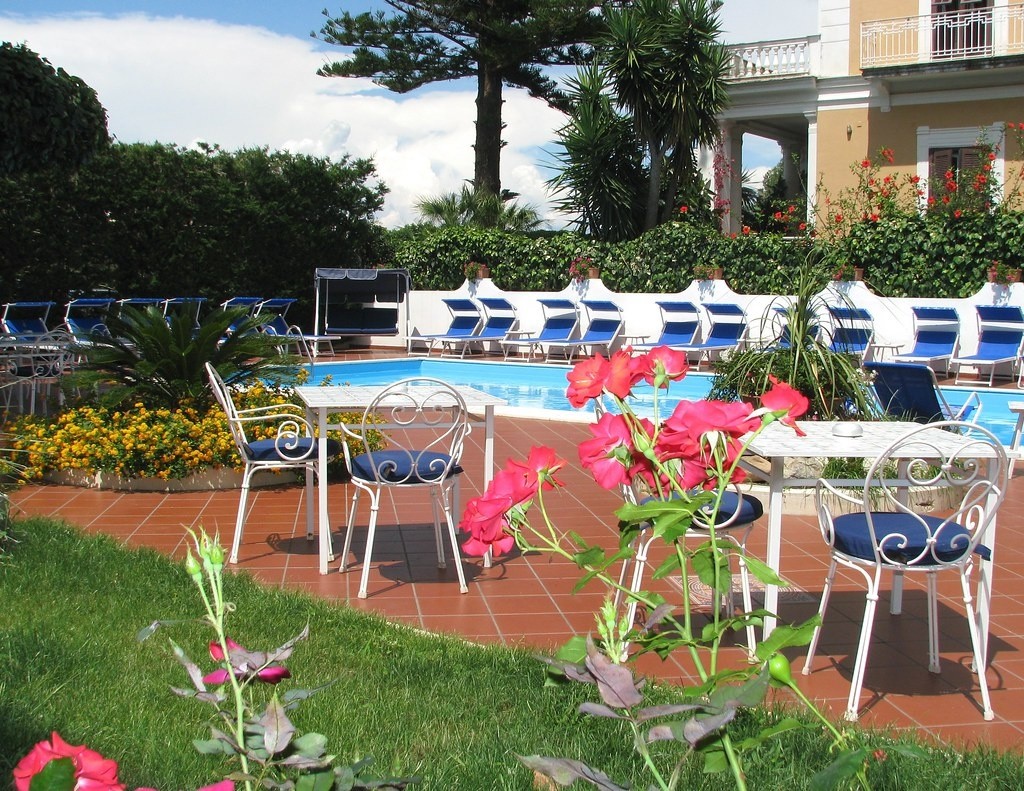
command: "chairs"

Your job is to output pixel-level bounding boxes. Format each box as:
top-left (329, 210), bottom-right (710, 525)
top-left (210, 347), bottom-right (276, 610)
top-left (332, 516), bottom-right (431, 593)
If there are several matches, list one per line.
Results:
top-left (593, 392), bottom-right (764, 665)
top-left (0, 267), bottom-right (1024, 424)
top-left (864, 361), bottom-right (983, 437)
top-left (205, 361), bottom-right (341, 565)
top-left (801, 420), bottom-right (1009, 722)
top-left (338, 377), bottom-right (473, 599)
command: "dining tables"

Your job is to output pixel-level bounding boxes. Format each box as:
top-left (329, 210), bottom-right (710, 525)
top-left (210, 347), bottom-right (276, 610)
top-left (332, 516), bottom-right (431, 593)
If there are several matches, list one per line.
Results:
top-left (294, 385), bottom-right (509, 576)
top-left (709, 422), bottom-right (1021, 673)
